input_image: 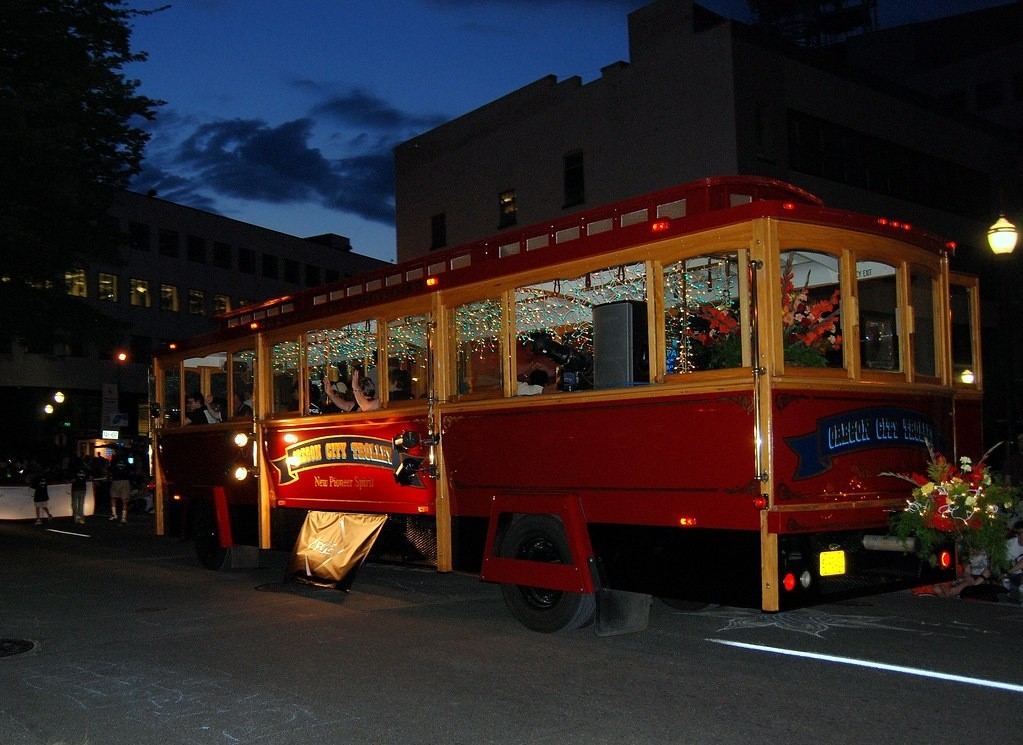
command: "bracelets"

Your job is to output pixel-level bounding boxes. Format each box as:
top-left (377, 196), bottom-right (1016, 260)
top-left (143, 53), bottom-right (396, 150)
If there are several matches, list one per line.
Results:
top-left (353, 389), bottom-right (360, 392)
top-left (330, 394), bottom-right (336, 399)
top-left (980, 575), bottom-right (986, 580)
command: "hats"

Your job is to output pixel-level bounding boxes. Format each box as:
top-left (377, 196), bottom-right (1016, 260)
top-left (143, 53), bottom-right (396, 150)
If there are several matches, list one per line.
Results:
top-left (331, 382), bottom-right (347, 393)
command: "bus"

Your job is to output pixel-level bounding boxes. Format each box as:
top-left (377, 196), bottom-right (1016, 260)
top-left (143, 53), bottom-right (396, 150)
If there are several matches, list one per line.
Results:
top-left (77, 437), bottom-right (133, 466)
top-left (151, 179), bottom-right (984, 634)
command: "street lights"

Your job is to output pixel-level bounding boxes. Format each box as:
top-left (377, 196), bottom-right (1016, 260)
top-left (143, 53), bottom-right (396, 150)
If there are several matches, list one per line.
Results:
top-left (989, 210), bottom-right (1023, 479)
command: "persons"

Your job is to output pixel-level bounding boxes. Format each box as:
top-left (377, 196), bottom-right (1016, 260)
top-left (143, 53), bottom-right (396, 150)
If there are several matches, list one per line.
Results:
top-left (107, 447), bottom-right (130, 524)
top-left (30, 471), bottom-right (52, 524)
top-left (353, 370), bottom-right (414, 411)
top-left (517, 369), bottom-right (559, 395)
top-left (184, 384), bottom-right (253, 426)
top-left (323, 377), bottom-right (375, 412)
top-left (65, 459), bottom-right (93, 522)
top-left (933, 521), bottom-right (1023, 598)
top-left (274, 374), bottom-right (346, 414)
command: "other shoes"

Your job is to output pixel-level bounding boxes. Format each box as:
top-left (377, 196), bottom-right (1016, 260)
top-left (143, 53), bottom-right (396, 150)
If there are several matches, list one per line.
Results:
top-left (35, 519), bottom-right (42, 524)
top-left (120, 518), bottom-right (127, 523)
top-left (48, 515), bottom-right (52, 523)
top-left (75, 516), bottom-right (85, 524)
top-left (108, 514), bottom-right (119, 520)
